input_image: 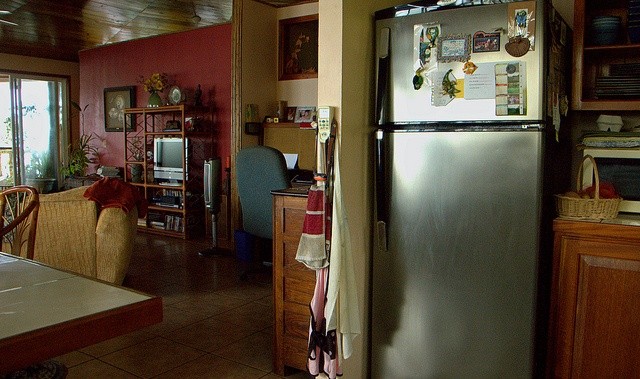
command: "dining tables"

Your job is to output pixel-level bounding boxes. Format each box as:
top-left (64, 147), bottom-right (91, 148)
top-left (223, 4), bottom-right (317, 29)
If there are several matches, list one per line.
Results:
top-left (3, 105), bottom-right (38, 190)
top-left (24, 151), bottom-right (56, 194)
top-left (60, 103), bottom-right (99, 181)
top-left (127, 139), bottom-right (145, 183)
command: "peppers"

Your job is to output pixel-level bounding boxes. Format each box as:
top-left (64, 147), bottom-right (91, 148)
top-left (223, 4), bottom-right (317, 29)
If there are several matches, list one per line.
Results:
top-left (591, 189), bottom-right (596, 198)
top-left (581, 195), bottom-right (590, 198)
top-left (563, 191), bottom-right (580, 198)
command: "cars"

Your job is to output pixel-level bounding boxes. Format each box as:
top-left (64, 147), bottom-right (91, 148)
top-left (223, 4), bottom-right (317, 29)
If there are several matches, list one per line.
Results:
top-left (626, 0), bottom-right (640, 44)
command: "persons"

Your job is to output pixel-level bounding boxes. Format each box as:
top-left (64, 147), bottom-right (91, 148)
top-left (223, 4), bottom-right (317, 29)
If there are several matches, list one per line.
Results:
top-left (298, 109), bottom-right (311, 121)
top-left (288, 107), bottom-right (294, 121)
top-left (109, 94), bottom-right (128, 123)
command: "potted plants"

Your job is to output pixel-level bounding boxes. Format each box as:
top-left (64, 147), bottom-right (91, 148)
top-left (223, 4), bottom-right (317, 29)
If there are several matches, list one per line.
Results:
top-left (103, 86), bottom-right (137, 133)
top-left (294, 106), bottom-right (314, 123)
top-left (277, 14), bottom-right (318, 82)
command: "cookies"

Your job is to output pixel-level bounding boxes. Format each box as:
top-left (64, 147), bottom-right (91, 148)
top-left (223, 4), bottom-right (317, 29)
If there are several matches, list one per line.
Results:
top-left (582, 149), bottom-right (640, 216)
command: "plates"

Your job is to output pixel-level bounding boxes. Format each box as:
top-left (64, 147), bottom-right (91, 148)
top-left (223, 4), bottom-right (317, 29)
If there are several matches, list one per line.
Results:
top-left (550, 222), bottom-right (639, 378)
top-left (271, 195), bottom-right (315, 377)
top-left (123, 104), bottom-right (216, 238)
top-left (575, 2), bottom-right (638, 110)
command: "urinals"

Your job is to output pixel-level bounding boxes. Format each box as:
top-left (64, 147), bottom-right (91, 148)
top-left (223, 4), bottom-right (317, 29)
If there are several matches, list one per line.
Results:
top-left (455, 79), bottom-right (464, 98)
top-left (432, 70), bottom-right (455, 106)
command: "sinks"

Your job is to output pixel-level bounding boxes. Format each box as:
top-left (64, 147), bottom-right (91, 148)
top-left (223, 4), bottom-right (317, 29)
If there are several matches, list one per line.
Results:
top-left (18, 177), bottom-right (148, 285)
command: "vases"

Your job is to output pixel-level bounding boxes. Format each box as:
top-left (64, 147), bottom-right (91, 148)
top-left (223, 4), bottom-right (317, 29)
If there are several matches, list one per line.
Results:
top-left (142, 71), bottom-right (169, 93)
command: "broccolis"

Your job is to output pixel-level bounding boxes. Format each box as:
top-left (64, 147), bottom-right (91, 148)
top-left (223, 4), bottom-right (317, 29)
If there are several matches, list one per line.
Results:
top-left (370, 0), bottom-right (574, 379)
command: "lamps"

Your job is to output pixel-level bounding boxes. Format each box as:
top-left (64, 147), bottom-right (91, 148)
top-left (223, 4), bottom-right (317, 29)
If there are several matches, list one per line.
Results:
top-left (0, 185), bottom-right (41, 260)
top-left (235, 146), bottom-right (290, 282)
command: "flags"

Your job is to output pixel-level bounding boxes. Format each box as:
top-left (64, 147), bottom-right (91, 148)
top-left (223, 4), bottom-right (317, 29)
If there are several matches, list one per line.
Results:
top-left (146, 92), bottom-right (163, 107)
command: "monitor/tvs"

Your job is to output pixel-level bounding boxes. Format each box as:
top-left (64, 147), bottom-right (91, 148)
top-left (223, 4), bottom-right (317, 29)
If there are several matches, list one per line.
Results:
top-left (162, 189), bottom-right (184, 205)
top-left (137, 216), bottom-right (146, 227)
top-left (148, 210), bottom-right (184, 233)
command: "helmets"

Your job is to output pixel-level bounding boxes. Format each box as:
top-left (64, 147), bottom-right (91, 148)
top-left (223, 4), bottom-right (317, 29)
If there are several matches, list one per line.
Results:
top-left (591, 15), bottom-right (621, 46)
top-left (593, 63), bottom-right (640, 100)
